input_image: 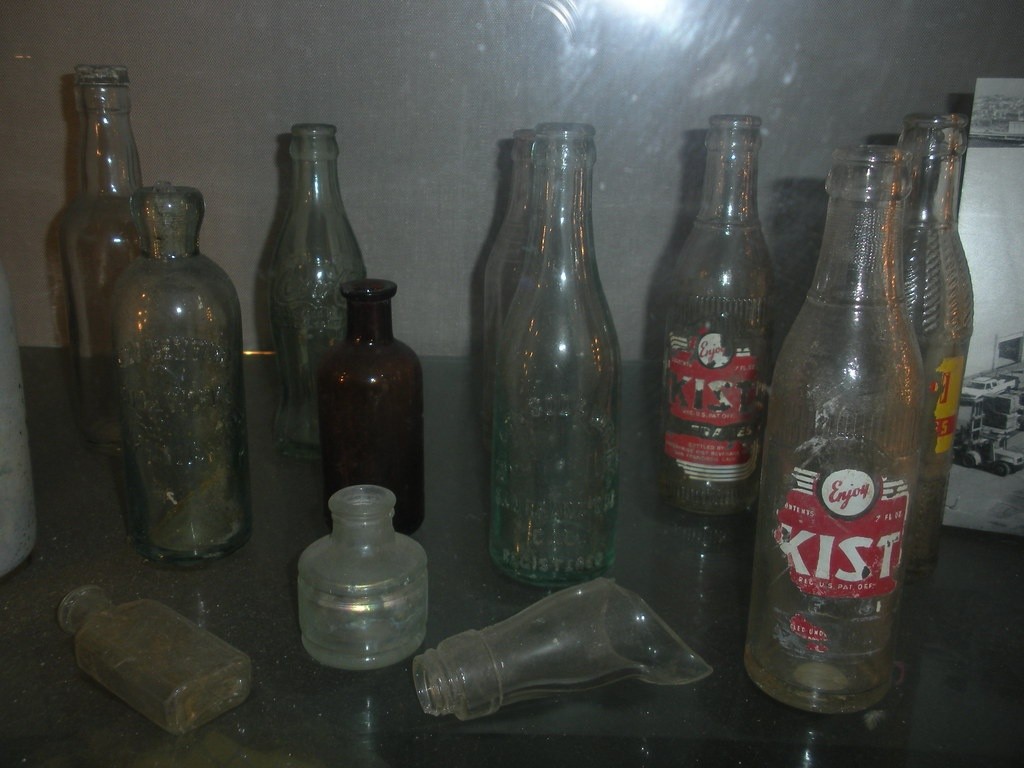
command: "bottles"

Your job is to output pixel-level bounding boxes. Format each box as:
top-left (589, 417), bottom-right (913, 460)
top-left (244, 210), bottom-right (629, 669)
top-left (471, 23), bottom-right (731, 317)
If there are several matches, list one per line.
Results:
top-left (742, 144), bottom-right (926, 713)
top-left (896, 112), bottom-right (975, 562)
top-left (0, 260), bottom-right (37, 579)
top-left (58, 63), bottom-right (143, 456)
top-left (657, 115), bottom-right (772, 516)
top-left (479, 122), bottom-right (621, 591)
top-left (56, 584), bottom-right (254, 736)
top-left (109, 180), bottom-right (254, 566)
top-left (319, 278), bottom-right (425, 537)
top-left (268, 122), bottom-right (368, 456)
top-left (295, 484), bottom-right (430, 672)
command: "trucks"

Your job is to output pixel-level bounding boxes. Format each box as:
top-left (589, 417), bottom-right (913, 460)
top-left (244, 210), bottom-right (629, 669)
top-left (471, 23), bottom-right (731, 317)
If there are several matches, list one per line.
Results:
top-left (951, 396), bottom-right (1024, 477)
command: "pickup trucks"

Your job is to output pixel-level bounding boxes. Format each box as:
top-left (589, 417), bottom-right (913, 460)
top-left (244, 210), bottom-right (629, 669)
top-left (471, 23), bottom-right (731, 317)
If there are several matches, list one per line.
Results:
top-left (960, 375), bottom-right (1017, 401)
